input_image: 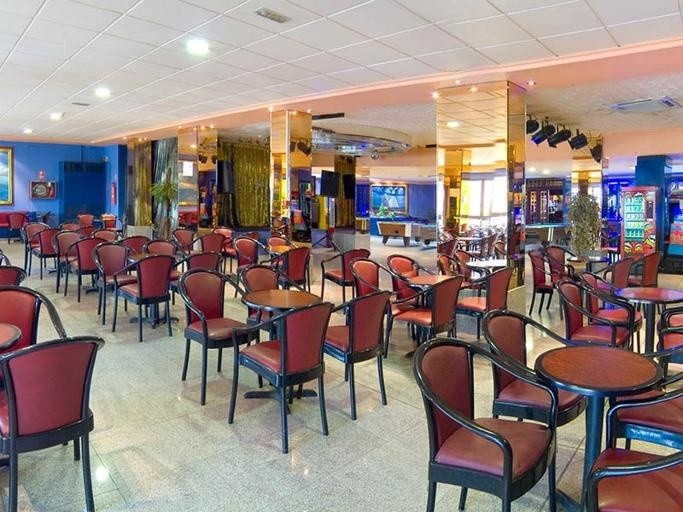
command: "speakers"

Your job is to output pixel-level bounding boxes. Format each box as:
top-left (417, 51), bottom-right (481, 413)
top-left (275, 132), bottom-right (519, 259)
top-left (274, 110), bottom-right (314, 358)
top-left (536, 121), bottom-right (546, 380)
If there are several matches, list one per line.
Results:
top-left (320, 170), bottom-right (340, 198)
top-left (342, 174), bottom-right (355, 200)
top-left (216, 160), bottom-right (234, 194)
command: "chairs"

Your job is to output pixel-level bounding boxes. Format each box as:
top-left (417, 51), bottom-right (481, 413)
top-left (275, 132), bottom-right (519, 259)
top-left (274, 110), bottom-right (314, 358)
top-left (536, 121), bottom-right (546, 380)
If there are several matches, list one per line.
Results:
top-left (226, 301), bottom-right (329, 454)
top-left (588, 389), bottom-right (682, 511)
top-left (657, 307), bottom-right (682, 378)
top-left (179, 269), bottom-right (263, 405)
top-left (1, 336), bottom-right (104, 512)
top-left (324, 291), bottom-right (392, 421)
top-left (609, 345), bottom-right (682, 451)
top-left (482, 308), bottom-right (589, 426)
top-left (413, 339), bottom-right (556, 512)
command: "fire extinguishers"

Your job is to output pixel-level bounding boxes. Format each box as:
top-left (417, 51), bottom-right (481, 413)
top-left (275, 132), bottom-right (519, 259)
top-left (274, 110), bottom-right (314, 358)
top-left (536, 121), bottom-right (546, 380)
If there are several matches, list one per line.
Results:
top-left (111, 182), bottom-right (116, 205)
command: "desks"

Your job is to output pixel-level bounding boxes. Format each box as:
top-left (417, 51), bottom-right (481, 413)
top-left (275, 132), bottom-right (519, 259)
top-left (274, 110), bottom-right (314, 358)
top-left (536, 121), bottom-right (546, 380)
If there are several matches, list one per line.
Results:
top-left (532, 344), bottom-right (662, 512)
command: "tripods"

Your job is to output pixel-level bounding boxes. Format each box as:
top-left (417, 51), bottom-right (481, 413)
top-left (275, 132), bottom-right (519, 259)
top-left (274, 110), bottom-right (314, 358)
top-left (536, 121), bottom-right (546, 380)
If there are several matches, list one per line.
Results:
top-left (311, 197), bottom-right (342, 253)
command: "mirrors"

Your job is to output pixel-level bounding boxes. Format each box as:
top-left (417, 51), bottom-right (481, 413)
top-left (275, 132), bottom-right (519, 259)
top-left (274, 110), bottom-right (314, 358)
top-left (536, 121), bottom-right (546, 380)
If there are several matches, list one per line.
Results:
top-left (269, 112), bottom-right (287, 232)
top-left (571, 171), bottom-right (601, 249)
top-left (435, 81), bottom-right (508, 265)
top-left (508, 82), bottom-right (526, 271)
top-left (290, 108), bottom-right (311, 219)
top-left (177, 128), bottom-right (199, 229)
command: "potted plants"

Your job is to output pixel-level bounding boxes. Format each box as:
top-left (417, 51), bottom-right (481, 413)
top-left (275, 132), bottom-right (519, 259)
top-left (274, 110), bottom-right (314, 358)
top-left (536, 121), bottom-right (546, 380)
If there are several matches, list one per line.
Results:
top-left (562, 190), bottom-right (607, 274)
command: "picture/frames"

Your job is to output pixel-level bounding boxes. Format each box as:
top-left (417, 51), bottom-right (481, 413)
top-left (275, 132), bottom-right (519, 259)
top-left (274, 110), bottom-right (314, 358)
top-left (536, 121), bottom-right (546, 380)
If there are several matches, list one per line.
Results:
top-left (0, 147), bottom-right (13, 205)
top-left (30, 180), bottom-right (56, 200)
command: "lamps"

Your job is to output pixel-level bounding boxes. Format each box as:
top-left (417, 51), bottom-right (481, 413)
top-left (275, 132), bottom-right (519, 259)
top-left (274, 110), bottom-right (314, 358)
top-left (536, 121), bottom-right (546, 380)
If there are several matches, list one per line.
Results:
top-left (526, 112), bottom-right (603, 163)
top-left (290, 138), bottom-right (311, 157)
